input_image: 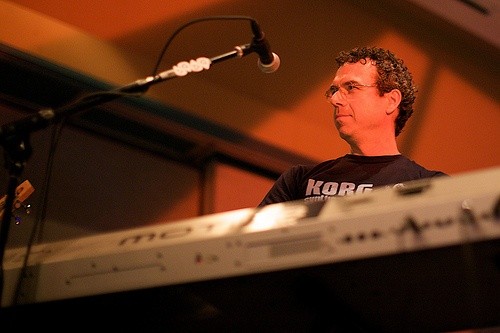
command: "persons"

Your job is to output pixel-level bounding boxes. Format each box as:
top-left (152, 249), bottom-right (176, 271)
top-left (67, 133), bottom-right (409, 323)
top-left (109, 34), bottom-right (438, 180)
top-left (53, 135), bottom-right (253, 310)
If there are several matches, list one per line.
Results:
top-left (209, 45), bottom-right (458, 333)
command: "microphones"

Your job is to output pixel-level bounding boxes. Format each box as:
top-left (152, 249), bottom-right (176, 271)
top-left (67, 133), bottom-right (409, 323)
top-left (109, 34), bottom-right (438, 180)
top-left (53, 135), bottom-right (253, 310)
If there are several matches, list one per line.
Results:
top-left (250, 20), bottom-right (280, 73)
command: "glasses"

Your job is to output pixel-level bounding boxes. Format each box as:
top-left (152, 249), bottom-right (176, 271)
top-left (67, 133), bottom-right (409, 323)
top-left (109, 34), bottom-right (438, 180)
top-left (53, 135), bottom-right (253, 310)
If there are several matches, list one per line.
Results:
top-left (324, 82), bottom-right (379, 103)
